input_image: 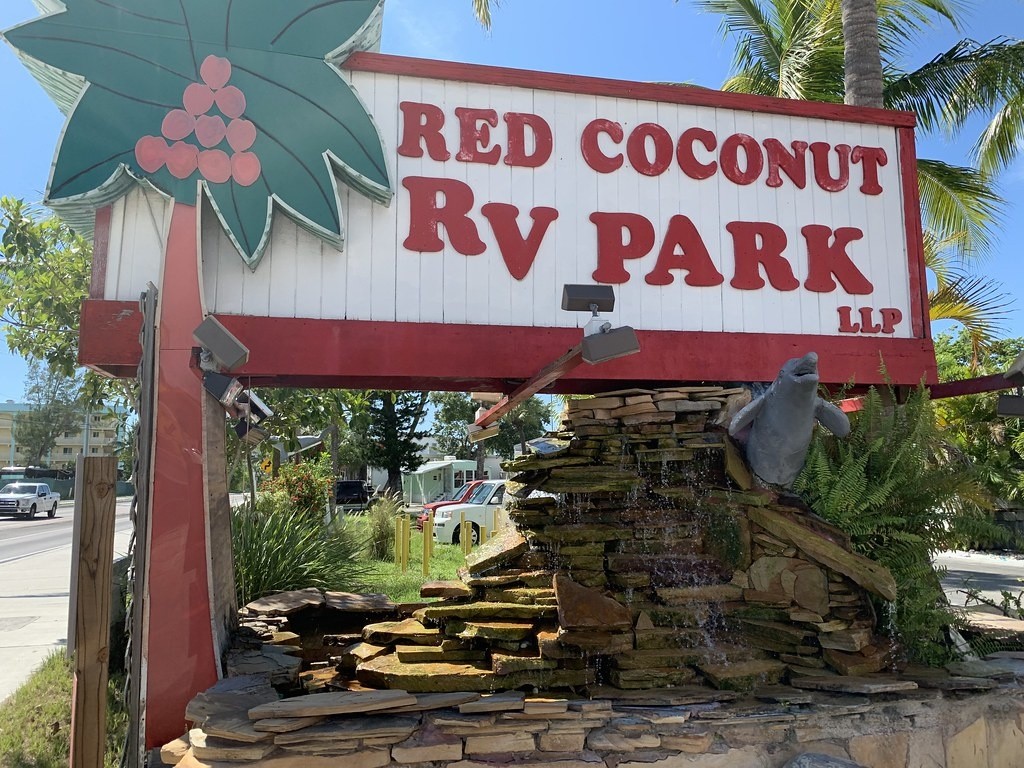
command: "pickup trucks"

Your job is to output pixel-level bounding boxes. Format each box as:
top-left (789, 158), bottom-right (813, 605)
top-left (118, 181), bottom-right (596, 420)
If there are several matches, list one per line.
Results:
top-left (0, 483), bottom-right (60, 521)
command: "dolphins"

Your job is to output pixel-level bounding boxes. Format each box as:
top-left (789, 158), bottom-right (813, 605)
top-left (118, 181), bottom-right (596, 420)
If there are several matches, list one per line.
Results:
top-left (729, 351), bottom-right (851, 491)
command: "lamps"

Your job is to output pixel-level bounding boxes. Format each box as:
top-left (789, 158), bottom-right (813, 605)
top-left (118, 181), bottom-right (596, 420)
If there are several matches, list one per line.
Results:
top-left (233, 420), bottom-right (270, 447)
top-left (237, 388), bottom-right (274, 426)
top-left (467, 421), bottom-right (500, 443)
top-left (192, 314), bottom-right (249, 372)
top-left (560, 283), bottom-right (615, 313)
top-left (581, 326), bottom-right (641, 365)
top-left (201, 372), bottom-right (244, 408)
top-left (996, 395), bottom-right (1024, 418)
top-left (470, 392), bottom-right (503, 405)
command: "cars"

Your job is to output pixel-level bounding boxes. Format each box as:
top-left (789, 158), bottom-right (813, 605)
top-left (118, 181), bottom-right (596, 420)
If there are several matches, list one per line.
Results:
top-left (433, 480), bottom-right (560, 547)
top-left (417, 480), bottom-right (484, 533)
top-left (336, 480), bottom-right (370, 511)
